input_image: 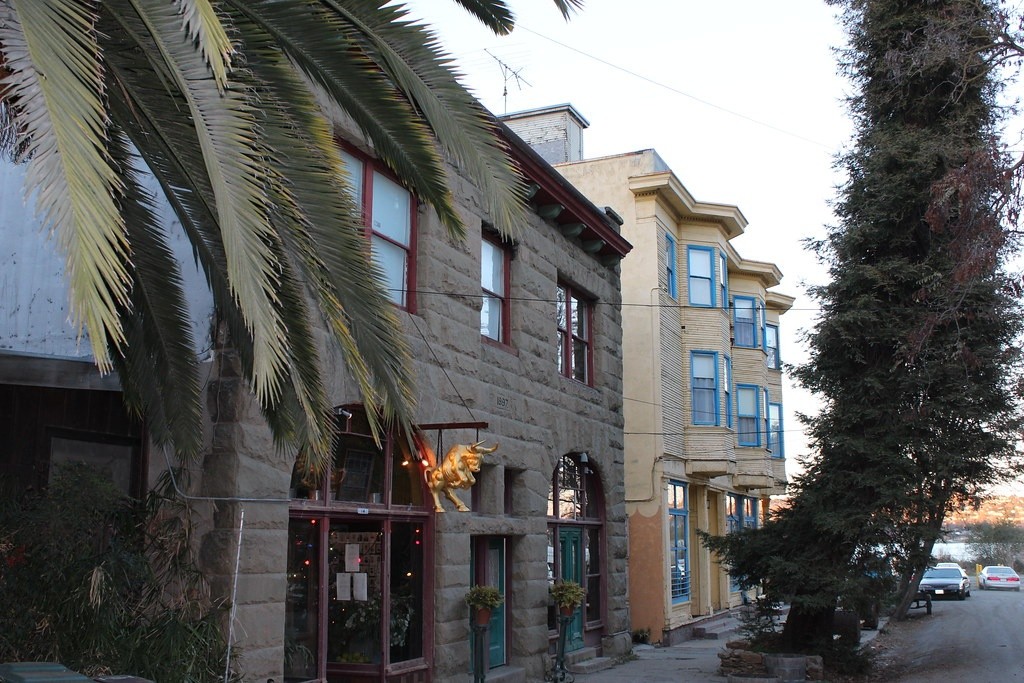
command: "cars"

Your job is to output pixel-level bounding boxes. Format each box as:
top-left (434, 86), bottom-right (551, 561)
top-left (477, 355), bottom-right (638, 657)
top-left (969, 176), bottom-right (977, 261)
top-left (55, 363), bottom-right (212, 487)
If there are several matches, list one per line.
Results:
top-left (670, 564), bottom-right (685, 595)
top-left (977, 565), bottom-right (1021, 591)
top-left (917, 563), bottom-right (971, 601)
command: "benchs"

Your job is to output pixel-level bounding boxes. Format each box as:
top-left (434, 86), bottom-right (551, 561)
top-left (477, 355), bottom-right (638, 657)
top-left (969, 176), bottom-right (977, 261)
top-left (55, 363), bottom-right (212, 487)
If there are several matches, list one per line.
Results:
top-left (754, 594), bottom-right (791, 622)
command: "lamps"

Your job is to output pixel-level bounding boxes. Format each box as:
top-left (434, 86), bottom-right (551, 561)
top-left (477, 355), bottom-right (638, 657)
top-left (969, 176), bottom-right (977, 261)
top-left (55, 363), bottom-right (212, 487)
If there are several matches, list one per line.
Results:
top-left (333, 408), bottom-right (352, 420)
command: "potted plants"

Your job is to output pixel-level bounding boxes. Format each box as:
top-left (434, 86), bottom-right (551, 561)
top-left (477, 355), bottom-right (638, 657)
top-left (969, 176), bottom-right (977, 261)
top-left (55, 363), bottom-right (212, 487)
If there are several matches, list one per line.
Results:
top-left (547, 577), bottom-right (586, 616)
top-left (464, 584), bottom-right (506, 629)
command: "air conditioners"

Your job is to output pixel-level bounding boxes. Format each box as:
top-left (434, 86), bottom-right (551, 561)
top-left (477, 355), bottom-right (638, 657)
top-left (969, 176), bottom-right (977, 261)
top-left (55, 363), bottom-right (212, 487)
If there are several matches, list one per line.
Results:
top-left (579, 453), bottom-right (588, 462)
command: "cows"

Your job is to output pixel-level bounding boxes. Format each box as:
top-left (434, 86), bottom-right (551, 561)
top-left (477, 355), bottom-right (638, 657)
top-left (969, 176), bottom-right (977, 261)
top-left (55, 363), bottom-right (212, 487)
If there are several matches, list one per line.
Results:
top-left (423, 438), bottom-right (500, 514)
